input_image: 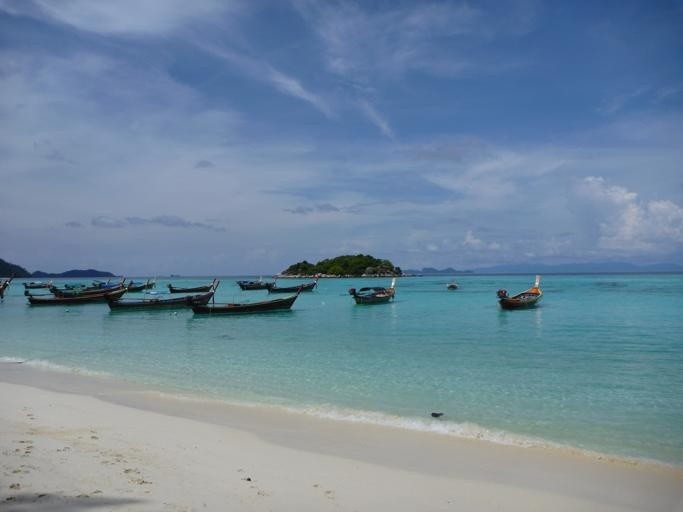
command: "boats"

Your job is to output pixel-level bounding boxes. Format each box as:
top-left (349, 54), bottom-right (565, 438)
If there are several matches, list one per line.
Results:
top-left (496, 275), bottom-right (543, 310)
top-left (348, 277), bottom-right (396, 304)
top-left (447, 283), bottom-right (458, 291)
